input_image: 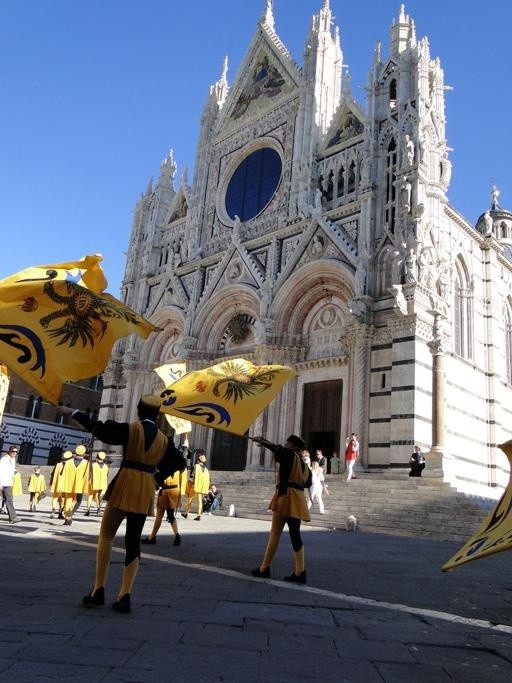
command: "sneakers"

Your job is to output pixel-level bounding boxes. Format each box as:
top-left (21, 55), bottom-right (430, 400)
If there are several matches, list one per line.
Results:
top-left (194, 516), bottom-right (199, 520)
top-left (251, 568), bottom-right (270, 578)
top-left (82, 587), bottom-right (104, 608)
top-left (113, 594), bottom-right (130, 613)
top-left (285, 571), bottom-right (306, 583)
top-left (9, 518), bottom-right (21, 524)
top-left (141, 538), bottom-right (156, 544)
top-left (182, 514), bottom-right (187, 518)
top-left (174, 533), bottom-right (180, 546)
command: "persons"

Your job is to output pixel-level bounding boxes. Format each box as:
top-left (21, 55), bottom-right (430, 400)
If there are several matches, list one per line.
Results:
top-left (301, 449), bottom-right (311, 471)
top-left (330, 453), bottom-right (340, 474)
top-left (0, 446), bottom-right (23, 524)
top-left (181, 454), bottom-right (210, 522)
top-left (56, 445), bottom-right (91, 526)
top-left (55, 394), bottom-right (187, 614)
top-left (344, 433), bottom-right (359, 481)
top-left (307, 461), bottom-right (325, 515)
top-left (84, 452), bottom-right (109, 516)
top-left (11, 465), bottom-right (23, 498)
top-left (139, 466), bottom-right (186, 547)
top-left (315, 450), bottom-right (330, 496)
top-left (203, 484), bottom-right (223, 516)
top-left (251, 435), bottom-right (313, 585)
top-left (409, 445), bottom-right (426, 477)
top-left (50, 450), bottom-right (73, 519)
top-left (27, 466), bottom-right (47, 512)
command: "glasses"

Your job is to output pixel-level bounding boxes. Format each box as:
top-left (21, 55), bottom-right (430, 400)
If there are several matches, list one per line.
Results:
top-left (12, 451), bottom-right (17, 453)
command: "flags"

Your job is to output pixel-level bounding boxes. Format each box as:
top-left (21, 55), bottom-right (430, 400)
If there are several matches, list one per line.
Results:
top-left (440, 440), bottom-right (512, 573)
top-left (1, 253), bottom-right (166, 407)
top-left (0, 359), bottom-right (12, 432)
top-left (138, 358), bottom-right (300, 437)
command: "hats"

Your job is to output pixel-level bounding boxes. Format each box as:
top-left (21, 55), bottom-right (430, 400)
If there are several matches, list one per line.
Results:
top-left (64, 451), bottom-right (72, 458)
top-left (288, 434), bottom-right (305, 449)
top-left (199, 455), bottom-right (206, 462)
top-left (76, 445), bottom-right (85, 454)
top-left (98, 452), bottom-right (106, 459)
top-left (139, 394), bottom-right (161, 411)
